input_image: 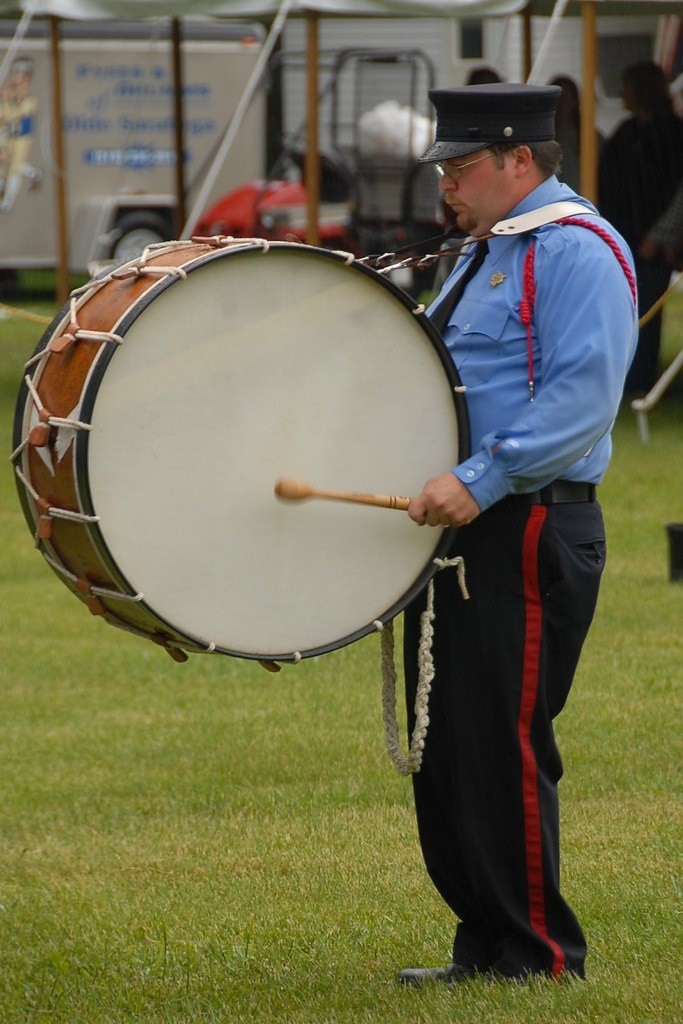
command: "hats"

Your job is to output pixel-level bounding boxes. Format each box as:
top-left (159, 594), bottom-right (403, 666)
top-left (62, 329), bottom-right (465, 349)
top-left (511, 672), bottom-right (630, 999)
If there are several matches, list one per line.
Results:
top-left (417, 83), bottom-right (561, 164)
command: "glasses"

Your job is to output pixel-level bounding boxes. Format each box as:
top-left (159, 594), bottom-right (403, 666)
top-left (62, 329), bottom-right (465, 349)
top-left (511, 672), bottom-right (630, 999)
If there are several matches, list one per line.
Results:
top-left (432, 152), bottom-right (502, 181)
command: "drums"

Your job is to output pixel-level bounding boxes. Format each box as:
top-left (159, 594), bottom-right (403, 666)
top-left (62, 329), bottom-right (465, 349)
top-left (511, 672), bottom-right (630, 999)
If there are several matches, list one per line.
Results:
top-left (8, 235), bottom-right (474, 674)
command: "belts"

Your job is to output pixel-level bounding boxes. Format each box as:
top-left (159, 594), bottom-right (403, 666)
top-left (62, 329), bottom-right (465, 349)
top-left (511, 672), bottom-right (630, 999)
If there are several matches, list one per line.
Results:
top-left (527, 479), bottom-right (596, 504)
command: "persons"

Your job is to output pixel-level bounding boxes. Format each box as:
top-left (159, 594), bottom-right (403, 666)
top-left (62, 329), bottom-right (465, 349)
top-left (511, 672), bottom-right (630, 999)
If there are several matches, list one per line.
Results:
top-left (392, 78), bottom-right (641, 987)
top-left (465, 60), bottom-right (683, 403)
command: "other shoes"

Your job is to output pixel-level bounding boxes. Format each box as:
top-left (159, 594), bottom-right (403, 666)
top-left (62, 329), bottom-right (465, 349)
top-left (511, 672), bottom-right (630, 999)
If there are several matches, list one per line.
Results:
top-left (397, 962), bottom-right (476, 989)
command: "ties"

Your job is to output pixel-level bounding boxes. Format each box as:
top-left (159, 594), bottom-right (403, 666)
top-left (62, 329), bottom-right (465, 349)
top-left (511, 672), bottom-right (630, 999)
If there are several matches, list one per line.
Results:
top-left (428, 239), bottom-right (490, 335)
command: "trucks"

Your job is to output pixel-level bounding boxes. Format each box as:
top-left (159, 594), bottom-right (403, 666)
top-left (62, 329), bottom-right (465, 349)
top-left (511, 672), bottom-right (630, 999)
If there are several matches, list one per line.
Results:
top-left (0, 0), bottom-right (683, 303)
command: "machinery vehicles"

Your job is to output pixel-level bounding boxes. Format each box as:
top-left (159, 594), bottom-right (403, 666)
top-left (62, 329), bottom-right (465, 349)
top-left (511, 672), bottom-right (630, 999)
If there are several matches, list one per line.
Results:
top-left (190, 47), bottom-right (471, 300)
